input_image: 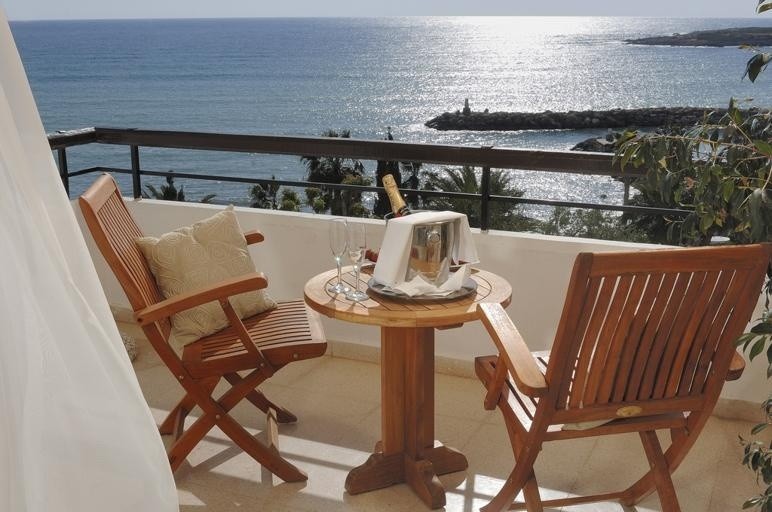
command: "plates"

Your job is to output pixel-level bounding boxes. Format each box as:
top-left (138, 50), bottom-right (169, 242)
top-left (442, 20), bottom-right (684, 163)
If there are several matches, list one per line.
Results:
top-left (366, 272), bottom-right (478, 302)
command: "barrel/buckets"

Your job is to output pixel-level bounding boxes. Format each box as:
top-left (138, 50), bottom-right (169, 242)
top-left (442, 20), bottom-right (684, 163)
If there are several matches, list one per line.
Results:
top-left (383, 210), bottom-right (450, 276)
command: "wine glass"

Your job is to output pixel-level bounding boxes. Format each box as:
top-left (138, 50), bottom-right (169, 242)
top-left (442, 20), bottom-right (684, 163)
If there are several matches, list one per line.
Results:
top-left (327, 217), bottom-right (381, 303)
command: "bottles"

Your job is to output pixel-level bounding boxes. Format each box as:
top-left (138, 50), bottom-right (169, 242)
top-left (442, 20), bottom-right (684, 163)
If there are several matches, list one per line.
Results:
top-left (381, 173), bottom-right (412, 219)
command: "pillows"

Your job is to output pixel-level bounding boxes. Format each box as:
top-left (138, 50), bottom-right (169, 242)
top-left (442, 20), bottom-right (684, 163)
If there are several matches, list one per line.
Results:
top-left (132, 203), bottom-right (279, 349)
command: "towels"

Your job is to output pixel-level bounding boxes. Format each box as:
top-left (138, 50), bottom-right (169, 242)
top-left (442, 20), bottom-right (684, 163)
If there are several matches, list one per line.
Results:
top-left (374, 210), bottom-right (480, 297)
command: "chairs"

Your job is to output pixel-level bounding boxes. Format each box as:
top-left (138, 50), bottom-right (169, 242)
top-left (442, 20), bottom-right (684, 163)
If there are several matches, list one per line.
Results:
top-left (474, 241), bottom-right (772, 512)
top-left (79, 172), bottom-right (328, 483)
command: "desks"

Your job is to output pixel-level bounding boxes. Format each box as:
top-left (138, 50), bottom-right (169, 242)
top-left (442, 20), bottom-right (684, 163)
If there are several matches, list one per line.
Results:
top-left (303, 264), bottom-right (512, 510)
